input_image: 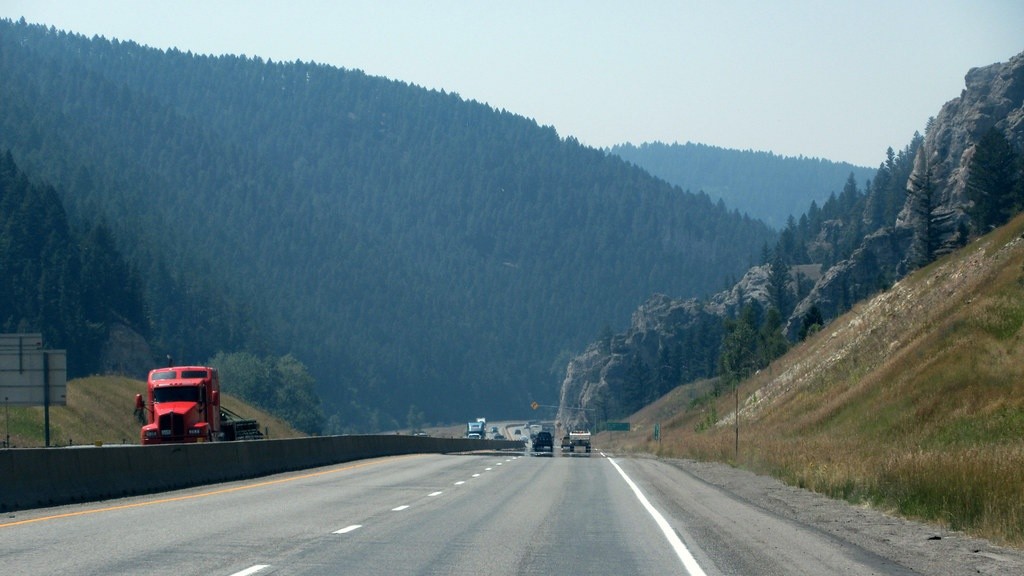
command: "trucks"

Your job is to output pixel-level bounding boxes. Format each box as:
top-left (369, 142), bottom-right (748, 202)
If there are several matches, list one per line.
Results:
top-left (570, 429), bottom-right (593, 454)
top-left (466, 417), bottom-right (487, 440)
top-left (131, 353), bottom-right (264, 446)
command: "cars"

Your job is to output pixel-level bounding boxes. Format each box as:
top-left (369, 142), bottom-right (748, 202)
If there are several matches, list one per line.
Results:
top-left (491, 425), bottom-right (505, 439)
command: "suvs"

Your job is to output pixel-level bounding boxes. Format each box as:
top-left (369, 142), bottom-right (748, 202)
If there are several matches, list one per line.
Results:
top-left (514, 420), bottom-right (555, 454)
top-left (560, 437), bottom-right (572, 451)
